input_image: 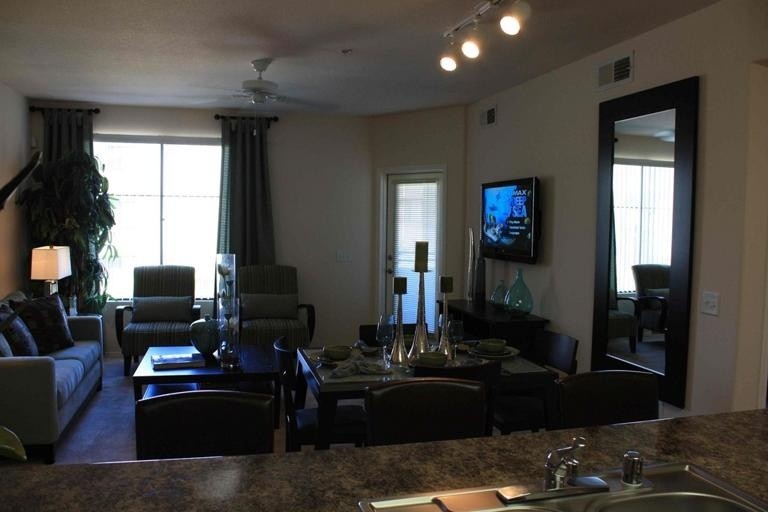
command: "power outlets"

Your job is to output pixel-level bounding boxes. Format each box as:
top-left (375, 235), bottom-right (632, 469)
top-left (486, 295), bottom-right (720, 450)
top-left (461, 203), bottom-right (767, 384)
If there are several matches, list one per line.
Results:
top-left (701, 289), bottom-right (721, 318)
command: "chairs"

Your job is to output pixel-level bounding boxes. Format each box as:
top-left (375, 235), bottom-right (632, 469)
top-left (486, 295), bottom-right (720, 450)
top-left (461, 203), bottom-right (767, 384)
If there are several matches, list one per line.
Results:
top-left (365, 376), bottom-right (489, 447)
top-left (115, 266), bottom-right (202, 376)
top-left (632, 265), bottom-right (672, 344)
top-left (235, 263), bottom-right (316, 346)
top-left (135, 389), bottom-right (274, 460)
top-left (553, 369), bottom-right (658, 429)
top-left (273, 335), bottom-right (366, 452)
top-left (608, 288), bottom-right (640, 353)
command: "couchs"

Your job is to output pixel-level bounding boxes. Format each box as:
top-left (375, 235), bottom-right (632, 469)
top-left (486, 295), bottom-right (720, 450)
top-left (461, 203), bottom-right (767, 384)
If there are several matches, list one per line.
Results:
top-left (0, 290), bottom-right (104, 448)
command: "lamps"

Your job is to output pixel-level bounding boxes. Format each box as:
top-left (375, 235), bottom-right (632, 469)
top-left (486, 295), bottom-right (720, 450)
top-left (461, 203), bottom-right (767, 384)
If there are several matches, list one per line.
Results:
top-left (440, 1), bottom-right (532, 72)
top-left (30, 245), bottom-right (72, 297)
top-left (252, 89), bottom-right (267, 106)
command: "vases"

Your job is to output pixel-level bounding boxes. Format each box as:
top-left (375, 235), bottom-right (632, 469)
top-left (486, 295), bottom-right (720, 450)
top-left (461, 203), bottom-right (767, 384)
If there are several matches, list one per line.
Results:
top-left (188, 314), bottom-right (224, 353)
top-left (504, 266), bottom-right (534, 316)
top-left (216, 253), bottom-right (240, 369)
top-left (488, 280), bottom-right (511, 312)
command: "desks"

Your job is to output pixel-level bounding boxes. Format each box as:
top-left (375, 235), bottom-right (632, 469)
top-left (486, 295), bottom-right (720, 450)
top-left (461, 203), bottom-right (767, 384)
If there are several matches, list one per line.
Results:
top-left (294, 340), bottom-right (558, 450)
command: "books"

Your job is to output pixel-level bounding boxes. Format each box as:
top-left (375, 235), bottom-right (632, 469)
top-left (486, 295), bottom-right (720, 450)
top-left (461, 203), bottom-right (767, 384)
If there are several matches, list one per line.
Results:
top-left (150, 351), bottom-right (207, 371)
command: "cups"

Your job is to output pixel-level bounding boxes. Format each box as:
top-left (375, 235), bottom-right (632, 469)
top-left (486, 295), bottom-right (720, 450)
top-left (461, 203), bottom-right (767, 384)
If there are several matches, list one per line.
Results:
top-left (438, 313), bottom-right (453, 333)
top-left (620, 448), bottom-right (645, 487)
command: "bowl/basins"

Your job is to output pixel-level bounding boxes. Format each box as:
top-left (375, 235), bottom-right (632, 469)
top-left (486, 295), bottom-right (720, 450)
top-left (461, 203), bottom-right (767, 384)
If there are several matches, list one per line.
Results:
top-left (478, 338), bottom-right (506, 352)
top-left (321, 345), bottom-right (350, 360)
top-left (416, 351), bottom-right (448, 365)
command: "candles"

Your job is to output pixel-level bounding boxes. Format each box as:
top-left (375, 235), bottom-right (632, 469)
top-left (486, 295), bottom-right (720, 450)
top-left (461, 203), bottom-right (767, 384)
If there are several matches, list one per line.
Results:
top-left (436, 275), bottom-right (456, 294)
top-left (390, 276), bottom-right (409, 293)
top-left (408, 242), bottom-right (434, 273)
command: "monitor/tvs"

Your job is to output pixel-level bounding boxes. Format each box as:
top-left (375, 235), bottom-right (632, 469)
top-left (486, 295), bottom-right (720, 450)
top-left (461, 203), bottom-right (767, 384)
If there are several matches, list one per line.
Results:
top-left (479, 176), bottom-right (539, 264)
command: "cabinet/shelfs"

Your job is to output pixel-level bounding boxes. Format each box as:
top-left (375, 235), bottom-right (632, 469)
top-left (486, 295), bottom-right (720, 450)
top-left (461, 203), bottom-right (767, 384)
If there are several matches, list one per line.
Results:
top-left (437, 297), bottom-right (550, 358)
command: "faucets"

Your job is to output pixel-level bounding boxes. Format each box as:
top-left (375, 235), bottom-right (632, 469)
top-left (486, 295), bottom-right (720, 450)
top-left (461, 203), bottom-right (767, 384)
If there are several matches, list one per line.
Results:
top-left (544, 435), bottom-right (588, 491)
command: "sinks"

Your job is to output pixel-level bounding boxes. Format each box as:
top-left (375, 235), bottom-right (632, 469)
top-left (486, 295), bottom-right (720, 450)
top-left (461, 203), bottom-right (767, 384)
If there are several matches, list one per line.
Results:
top-left (356, 461), bottom-right (768, 511)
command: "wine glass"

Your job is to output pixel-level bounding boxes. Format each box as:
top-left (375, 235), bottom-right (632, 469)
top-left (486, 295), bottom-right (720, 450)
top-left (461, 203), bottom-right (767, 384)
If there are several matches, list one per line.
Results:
top-left (446, 320), bottom-right (465, 359)
top-left (374, 314), bottom-right (393, 371)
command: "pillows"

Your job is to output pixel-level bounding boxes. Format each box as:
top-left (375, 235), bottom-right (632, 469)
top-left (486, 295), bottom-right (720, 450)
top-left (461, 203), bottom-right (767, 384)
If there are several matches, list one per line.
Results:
top-left (131, 296), bottom-right (192, 323)
top-left (8, 293), bottom-right (75, 356)
top-left (643, 287), bottom-right (670, 310)
top-left (0, 304), bottom-right (39, 357)
top-left (240, 293), bottom-right (298, 321)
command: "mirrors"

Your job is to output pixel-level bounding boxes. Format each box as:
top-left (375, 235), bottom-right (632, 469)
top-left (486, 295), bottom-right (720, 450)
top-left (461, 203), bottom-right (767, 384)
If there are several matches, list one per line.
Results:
top-left (590, 76), bottom-right (700, 410)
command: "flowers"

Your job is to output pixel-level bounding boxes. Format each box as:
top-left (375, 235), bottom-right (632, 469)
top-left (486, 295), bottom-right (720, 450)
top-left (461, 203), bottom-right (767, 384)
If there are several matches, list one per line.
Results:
top-left (217, 264), bottom-right (240, 363)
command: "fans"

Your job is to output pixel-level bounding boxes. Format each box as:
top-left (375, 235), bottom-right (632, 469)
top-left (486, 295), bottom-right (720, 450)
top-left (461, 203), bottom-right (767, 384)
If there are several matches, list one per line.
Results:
top-left (189, 58), bottom-right (338, 109)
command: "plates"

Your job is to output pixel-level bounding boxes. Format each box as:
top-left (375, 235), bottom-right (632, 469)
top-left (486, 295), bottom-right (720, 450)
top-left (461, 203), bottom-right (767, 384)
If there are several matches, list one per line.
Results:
top-left (411, 358), bottom-right (493, 369)
top-left (310, 349), bottom-right (362, 365)
top-left (467, 346), bottom-right (519, 358)
